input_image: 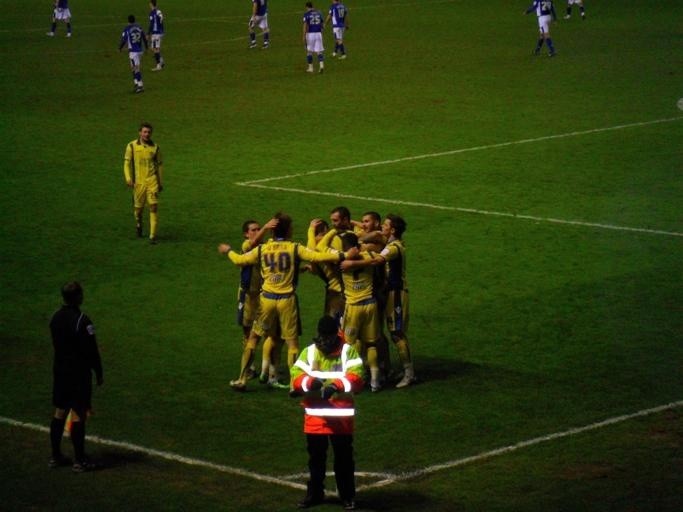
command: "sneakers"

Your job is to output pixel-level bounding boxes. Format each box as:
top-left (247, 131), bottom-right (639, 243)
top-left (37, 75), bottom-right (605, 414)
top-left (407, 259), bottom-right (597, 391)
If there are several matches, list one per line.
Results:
top-left (366, 369), bottom-right (416, 393)
top-left (150, 237), bottom-right (158, 244)
top-left (71, 460), bottom-right (104, 473)
top-left (48, 456), bottom-right (71, 468)
top-left (152, 63), bottom-right (166, 72)
top-left (296, 497), bottom-right (325, 507)
top-left (248, 42), bottom-right (258, 48)
top-left (134, 84), bottom-right (145, 94)
top-left (229, 370), bottom-right (291, 390)
top-left (305, 52), bottom-right (348, 73)
top-left (137, 222), bottom-right (144, 237)
top-left (45, 32), bottom-right (54, 37)
top-left (344, 499), bottom-right (354, 509)
top-left (261, 44), bottom-right (269, 49)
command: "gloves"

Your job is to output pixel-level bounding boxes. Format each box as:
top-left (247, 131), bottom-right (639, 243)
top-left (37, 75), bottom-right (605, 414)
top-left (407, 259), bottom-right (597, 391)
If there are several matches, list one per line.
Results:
top-left (321, 384), bottom-right (337, 400)
top-left (311, 378), bottom-right (326, 391)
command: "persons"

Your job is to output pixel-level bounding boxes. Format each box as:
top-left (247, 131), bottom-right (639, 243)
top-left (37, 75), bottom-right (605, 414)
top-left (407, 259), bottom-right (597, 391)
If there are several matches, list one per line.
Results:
top-left (48, 279), bottom-right (104, 473)
top-left (325, 0), bottom-right (349, 60)
top-left (45, 0), bottom-right (72, 38)
top-left (247, 0), bottom-right (270, 50)
top-left (289, 316), bottom-right (366, 510)
top-left (119, 15), bottom-right (148, 93)
top-left (145, 0), bottom-right (165, 71)
top-left (563, 0), bottom-right (585, 21)
top-left (302, 2), bottom-right (325, 73)
top-left (124, 121), bottom-right (163, 244)
top-left (523, 0), bottom-right (557, 57)
top-left (219, 206), bottom-right (416, 395)
